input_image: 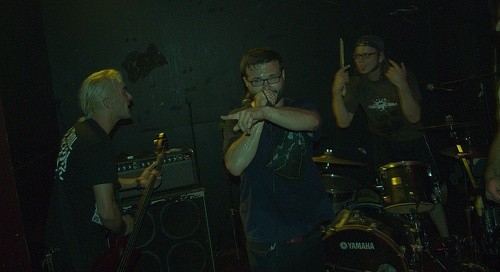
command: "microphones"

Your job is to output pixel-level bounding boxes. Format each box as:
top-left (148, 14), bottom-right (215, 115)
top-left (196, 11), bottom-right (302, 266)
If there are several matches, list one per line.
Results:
top-left (482, 206), bottom-right (500, 238)
top-left (444, 115), bottom-right (462, 145)
top-left (425, 83), bottom-right (454, 93)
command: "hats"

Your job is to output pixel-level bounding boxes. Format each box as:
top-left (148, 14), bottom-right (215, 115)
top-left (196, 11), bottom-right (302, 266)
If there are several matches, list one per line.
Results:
top-left (353, 34), bottom-right (384, 58)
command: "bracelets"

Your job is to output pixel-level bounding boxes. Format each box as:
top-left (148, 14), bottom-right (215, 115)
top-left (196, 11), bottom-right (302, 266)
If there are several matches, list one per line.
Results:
top-left (134, 177), bottom-right (143, 190)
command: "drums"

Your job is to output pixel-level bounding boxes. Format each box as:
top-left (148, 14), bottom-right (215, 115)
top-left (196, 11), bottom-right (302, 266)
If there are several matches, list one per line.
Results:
top-left (378, 162), bottom-right (436, 213)
top-left (447, 238), bottom-right (490, 272)
top-left (320, 205), bottom-right (413, 272)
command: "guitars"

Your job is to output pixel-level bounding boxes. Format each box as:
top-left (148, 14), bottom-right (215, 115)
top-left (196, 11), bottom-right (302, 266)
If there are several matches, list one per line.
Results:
top-left (94, 132), bottom-right (169, 272)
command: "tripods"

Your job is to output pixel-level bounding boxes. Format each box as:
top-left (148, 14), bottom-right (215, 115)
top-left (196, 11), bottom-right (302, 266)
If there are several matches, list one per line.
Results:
top-left (421, 136), bottom-right (479, 272)
top-left (216, 184), bottom-right (241, 262)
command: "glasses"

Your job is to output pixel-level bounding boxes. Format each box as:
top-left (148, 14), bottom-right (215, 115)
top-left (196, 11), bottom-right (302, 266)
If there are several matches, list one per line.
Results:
top-left (353, 52), bottom-right (378, 59)
top-left (245, 72), bottom-right (282, 87)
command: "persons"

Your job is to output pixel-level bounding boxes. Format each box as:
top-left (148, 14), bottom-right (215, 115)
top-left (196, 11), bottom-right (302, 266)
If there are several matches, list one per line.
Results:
top-left (333, 34), bottom-right (455, 242)
top-left (220, 48), bottom-right (333, 272)
top-left (482, 78), bottom-right (500, 202)
top-left (42, 68), bottom-right (163, 272)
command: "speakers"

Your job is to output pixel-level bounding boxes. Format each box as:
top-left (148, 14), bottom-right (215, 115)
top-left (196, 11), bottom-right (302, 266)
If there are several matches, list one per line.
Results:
top-left (120, 187), bottom-right (216, 272)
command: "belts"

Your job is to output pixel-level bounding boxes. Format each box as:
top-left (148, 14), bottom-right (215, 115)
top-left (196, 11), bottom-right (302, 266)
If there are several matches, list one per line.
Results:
top-left (283, 236), bottom-right (304, 244)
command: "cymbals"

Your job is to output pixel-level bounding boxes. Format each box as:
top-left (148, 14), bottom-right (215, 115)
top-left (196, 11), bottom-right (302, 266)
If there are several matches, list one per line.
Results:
top-left (312, 155), bottom-right (366, 166)
top-left (419, 120), bottom-right (481, 131)
top-left (438, 70), bottom-right (500, 86)
top-left (438, 145), bottom-right (489, 157)
